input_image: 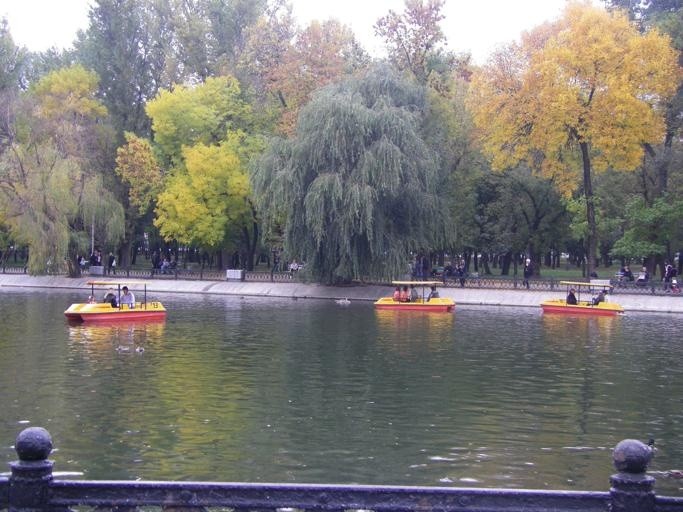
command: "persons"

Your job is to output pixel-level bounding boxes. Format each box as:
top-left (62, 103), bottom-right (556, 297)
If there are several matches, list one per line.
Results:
top-left (618, 265), bottom-right (634, 289)
top-left (103, 286), bottom-right (135, 309)
top-left (636, 266), bottom-right (649, 287)
top-left (426, 287), bottom-right (440, 302)
top-left (566, 289), bottom-right (578, 305)
top-left (289, 260), bottom-right (299, 277)
top-left (150, 251), bottom-right (178, 274)
top-left (522, 257), bottom-right (533, 290)
top-left (442, 255), bottom-right (468, 286)
top-left (75, 250), bottom-right (119, 278)
top-left (592, 290), bottom-right (606, 306)
top-left (392, 286), bottom-right (418, 303)
top-left (661, 261), bottom-right (675, 292)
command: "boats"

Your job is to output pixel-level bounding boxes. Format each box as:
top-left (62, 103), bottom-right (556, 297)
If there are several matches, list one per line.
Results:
top-left (374, 281), bottom-right (456, 311)
top-left (540, 281), bottom-right (625, 316)
top-left (63, 279), bottom-right (167, 324)
